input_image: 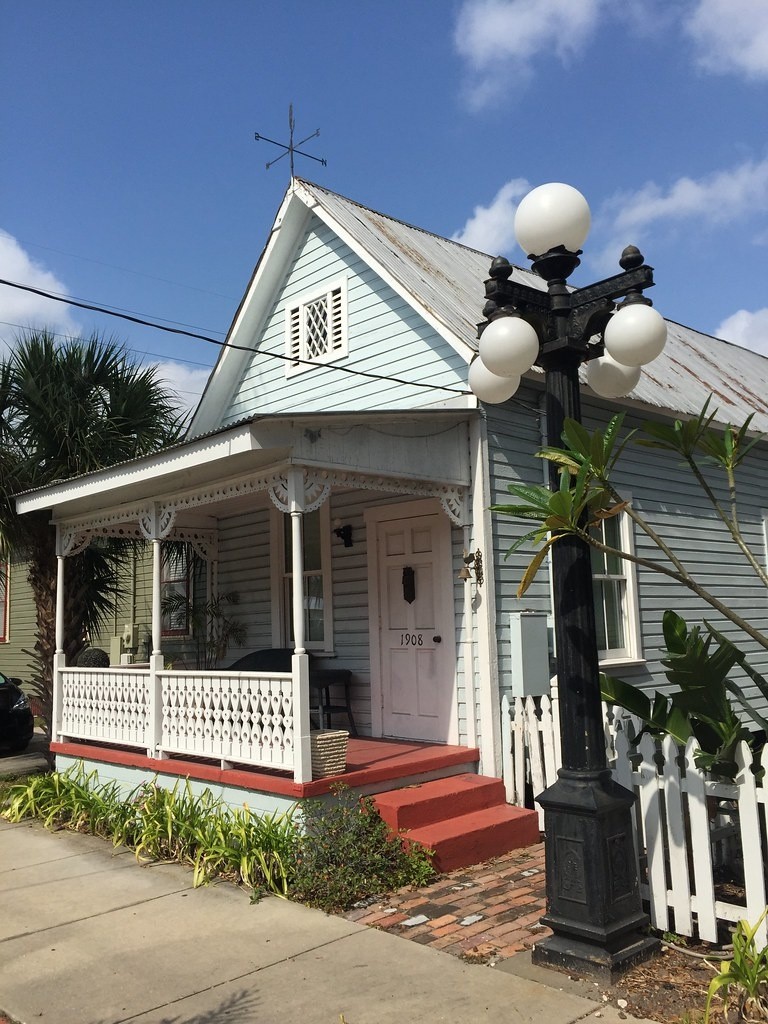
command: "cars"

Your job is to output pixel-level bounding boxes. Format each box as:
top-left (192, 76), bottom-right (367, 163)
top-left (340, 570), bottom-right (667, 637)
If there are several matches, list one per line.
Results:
top-left (0, 671), bottom-right (34, 751)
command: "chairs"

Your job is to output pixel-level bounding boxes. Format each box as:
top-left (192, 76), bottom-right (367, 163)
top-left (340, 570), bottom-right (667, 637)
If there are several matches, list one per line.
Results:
top-left (207, 649), bottom-right (320, 733)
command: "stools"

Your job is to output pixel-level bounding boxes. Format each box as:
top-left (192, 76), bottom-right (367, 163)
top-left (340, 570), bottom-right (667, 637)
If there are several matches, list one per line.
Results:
top-left (309, 669), bottom-right (359, 738)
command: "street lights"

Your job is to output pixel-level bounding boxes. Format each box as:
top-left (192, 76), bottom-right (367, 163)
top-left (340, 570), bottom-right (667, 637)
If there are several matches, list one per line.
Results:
top-left (469, 182), bottom-right (667, 989)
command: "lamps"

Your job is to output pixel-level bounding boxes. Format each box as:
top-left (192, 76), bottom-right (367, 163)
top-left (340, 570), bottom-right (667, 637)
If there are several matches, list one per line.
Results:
top-left (458, 545), bottom-right (485, 586)
top-left (332, 516), bottom-right (353, 547)
